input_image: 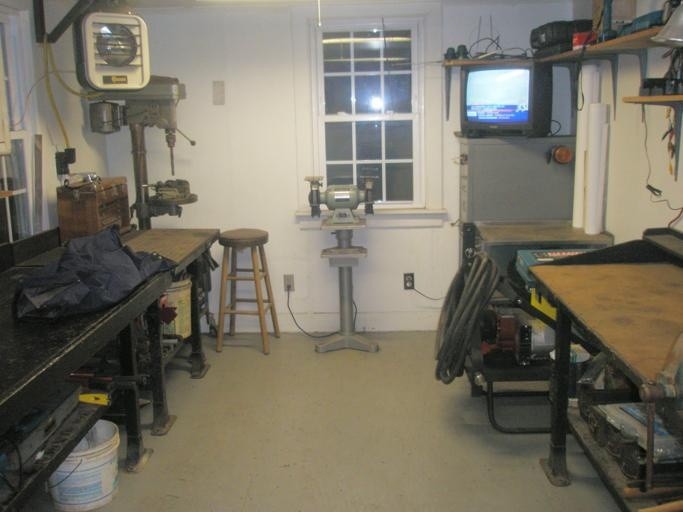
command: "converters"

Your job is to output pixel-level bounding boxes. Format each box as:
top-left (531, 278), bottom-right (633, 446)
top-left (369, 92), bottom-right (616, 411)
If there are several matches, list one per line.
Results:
top-left (65, 147), bottom-right (75, 165)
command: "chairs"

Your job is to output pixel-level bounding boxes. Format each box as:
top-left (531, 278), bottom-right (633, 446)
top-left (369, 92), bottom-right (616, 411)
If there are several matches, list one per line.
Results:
top-left (463, 250), bottom-right (600, 436)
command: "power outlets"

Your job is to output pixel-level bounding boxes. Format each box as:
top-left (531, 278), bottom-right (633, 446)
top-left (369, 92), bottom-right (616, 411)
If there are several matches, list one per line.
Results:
top-left (283, 275), bottom-right (295, 291)
top-left (404, 273), bottom-right (414, 289)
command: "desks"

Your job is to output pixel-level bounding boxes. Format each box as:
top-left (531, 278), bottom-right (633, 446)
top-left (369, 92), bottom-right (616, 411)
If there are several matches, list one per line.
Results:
top-left (528, 258), bottom-right (682, 512)
top-left (0, 268), bottom-right (178, 511)
top-left (13, 228), bottom-right (220, 402)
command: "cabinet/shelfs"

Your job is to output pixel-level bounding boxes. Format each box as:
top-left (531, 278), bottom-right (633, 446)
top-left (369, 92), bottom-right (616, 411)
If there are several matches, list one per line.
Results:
top-left (444, 26), bottom-right (682, 178)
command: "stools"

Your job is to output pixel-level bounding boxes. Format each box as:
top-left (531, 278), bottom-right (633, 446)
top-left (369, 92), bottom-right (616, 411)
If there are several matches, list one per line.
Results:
top-left (213, 227), bottom-right (283, 355)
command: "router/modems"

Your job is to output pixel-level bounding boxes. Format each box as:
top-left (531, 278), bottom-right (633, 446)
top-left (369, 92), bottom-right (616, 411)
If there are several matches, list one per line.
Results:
top-left (473, 15), bottom-right (527, 58)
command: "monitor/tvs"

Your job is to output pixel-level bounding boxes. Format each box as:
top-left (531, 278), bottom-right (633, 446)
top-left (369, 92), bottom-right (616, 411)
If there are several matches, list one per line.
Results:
top-left (530, 19), bottom-right (594, 59)
top-left (460, 62), bottom-right (552, 135)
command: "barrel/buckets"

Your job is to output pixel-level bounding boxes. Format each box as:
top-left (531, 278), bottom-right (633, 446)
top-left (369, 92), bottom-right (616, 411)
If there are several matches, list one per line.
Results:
top-left (47, 420), bottom-right (120, 511)
